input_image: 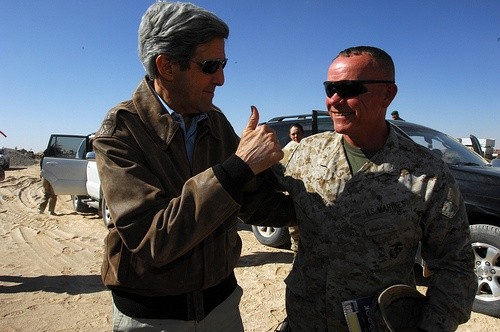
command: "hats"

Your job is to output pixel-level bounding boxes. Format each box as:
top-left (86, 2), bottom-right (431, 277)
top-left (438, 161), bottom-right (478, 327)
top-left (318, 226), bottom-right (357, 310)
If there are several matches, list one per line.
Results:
top-left (391, 110), bottom-right (398, 115)
top-left (371, 283), bottom-right (427, 332)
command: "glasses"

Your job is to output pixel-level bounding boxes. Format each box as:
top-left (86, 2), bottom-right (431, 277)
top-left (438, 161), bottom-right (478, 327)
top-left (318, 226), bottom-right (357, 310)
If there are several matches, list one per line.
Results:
top-left (177, 57), bottom-right (230, 74)
top-left (322, 79), bottom-right (396, 98)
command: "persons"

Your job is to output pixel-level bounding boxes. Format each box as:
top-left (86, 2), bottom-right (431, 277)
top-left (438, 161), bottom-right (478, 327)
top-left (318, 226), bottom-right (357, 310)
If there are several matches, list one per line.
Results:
top-left (261, 46), bottom-right (478, 332)
top-left (93, 0), bottom-right (296, 332)
top-left (278, 123), bottom-right (304, 265)
top-left (391, 111), bottom-right (403, 120)
top-left (38, 143), bottom-right (63, 215)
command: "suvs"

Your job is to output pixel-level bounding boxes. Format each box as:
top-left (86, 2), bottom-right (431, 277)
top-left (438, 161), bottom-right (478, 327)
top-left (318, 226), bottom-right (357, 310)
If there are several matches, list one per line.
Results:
top-left (40, 133), bottom-right (111, 226)
top-left (252, 110), bottom-right (500, 319)
